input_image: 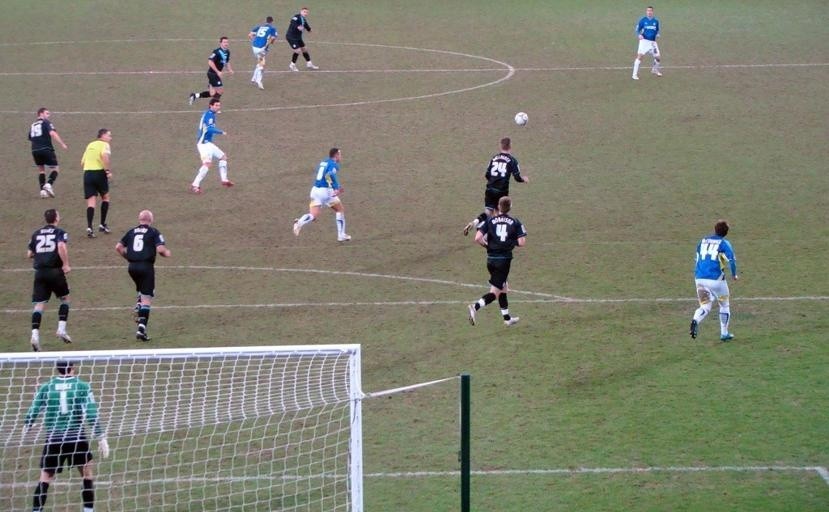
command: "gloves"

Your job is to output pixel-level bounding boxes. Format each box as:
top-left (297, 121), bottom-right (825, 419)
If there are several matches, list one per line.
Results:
top-left (97, 438), bottom-right (109, 459)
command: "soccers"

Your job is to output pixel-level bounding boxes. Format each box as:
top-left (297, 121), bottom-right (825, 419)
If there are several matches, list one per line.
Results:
top-left (514, 112), bottom-right (528, 127)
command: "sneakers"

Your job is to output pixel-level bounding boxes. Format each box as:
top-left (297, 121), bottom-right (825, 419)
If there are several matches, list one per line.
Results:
top-left (289, 62), bottom-right (298, 72)
top-left (135, 331), bottom-right (150, 341)
top-left (134, 304), bottom-right (141, 313)
top-left (43, 183), bottom-right (55, 198)
top-left (39, 190), bottom-right (50, 198)
top-left (189, 93), bottom-right (195, 106)
top-left (56, 331), bottom-right (72, 344)
top-left (251, 78), bottom-right (256, 83)
top-left (720, 334), bottom-right (734, 341)
top-left (463, 222), bottom-right (473, 236)
top-left (631, 74), bottom-right (639, 80)
top-left (191, 184), bottom-right (202, 194)
top-left (220, 181), bottom-right (235, 188)
top-left (504, 316), bottom-right (519, 326)
top-left (467, 304), bottom-right (477, 326)
top-left (99, 224), bottom-right (111, 233)
top-left (256, 80), bottom-right (264, 90)
top-left (306, 62), bottom-right (319, 70)
top-left (86, 227), bottom-right (96, 238)
top-left (30, 338), bottom-right (40, 351)
top-left (689, 319), bottom-right (698, 339)
top-left (651, 69), bottom-right (662, 77)
top-left (293, 218), bottom-right (301, 236)
top-left (337, 233), bottom-right (351, 242)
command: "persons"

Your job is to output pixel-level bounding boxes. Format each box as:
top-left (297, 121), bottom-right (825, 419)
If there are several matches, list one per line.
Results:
top-left (190, 98), bottom-right (235, 194)
top-left (28, 209), bottom-right (73, 352)
top-left (690, 221), bottom-right (737, 342)
top-left (189, 37), bottom-right (234, 113)
top-left (631, 6), bottom-right (663, 80)
top-left (22, 360), bottom-right (108, 512)
top-left (28, 107), bottom-right (69, 198)
top-left (462, 137), bottom-right (529, 236)
top-left (467, 196), bottom-right (530, 328)
top-left (248, 16), bottom-right (278, 91)
top-left (81, 128), bottom-right (114, 238)
top-left (294, 148), bottom-right (353, 243)
top-left (115, 210), bottom-right (172, 342)
top-left (286, 7), bottom-right (320, 71)
